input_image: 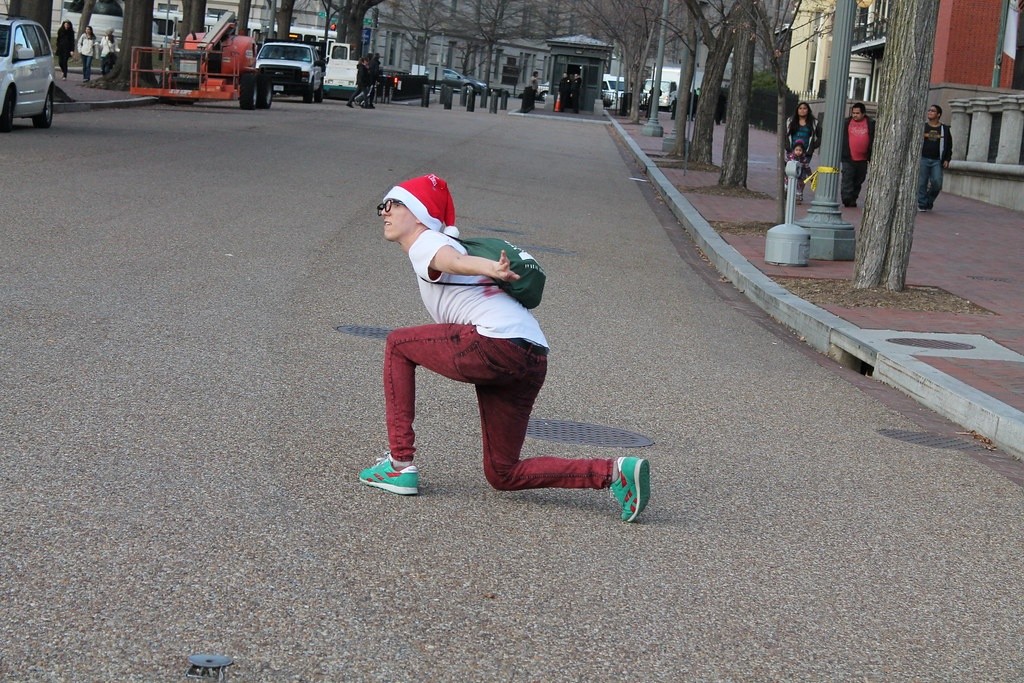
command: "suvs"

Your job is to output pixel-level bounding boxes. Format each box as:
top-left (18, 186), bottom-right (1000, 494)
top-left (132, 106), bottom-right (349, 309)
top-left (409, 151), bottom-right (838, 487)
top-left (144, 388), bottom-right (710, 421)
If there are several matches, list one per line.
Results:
top-left (0, 18), bottom-right (55, 128)
top-left (256, 41), bottom-right (324, 103)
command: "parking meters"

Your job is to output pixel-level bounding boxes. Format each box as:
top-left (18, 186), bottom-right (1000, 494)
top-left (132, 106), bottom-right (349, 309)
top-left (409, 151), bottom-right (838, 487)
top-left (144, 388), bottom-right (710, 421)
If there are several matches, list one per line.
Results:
top-left (512, 77), bottom-right (518, 97)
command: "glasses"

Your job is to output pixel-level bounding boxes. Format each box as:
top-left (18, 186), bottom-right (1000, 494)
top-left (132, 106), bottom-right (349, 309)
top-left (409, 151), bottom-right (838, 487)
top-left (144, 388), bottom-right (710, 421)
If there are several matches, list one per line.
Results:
top-left (928, 109), bottom-right (936, 112)
top-left (376, 199), bottom-right (405, 216)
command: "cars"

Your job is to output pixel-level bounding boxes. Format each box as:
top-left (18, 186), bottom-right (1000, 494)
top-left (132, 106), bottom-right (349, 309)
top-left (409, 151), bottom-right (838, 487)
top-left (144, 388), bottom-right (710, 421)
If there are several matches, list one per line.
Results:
top-left (381, 69), bottom-right (408, 78)
top-left (426, 68), bottom-right (487, 95)
top-left (535, 82), bottom-right (549, 101)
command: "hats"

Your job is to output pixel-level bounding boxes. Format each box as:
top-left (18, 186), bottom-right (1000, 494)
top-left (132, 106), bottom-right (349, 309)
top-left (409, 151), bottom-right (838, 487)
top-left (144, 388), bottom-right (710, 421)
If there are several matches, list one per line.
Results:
top-left (383, 174), bottom-right (459, 239)
top-left (105, 27), bottom-right (114, 34)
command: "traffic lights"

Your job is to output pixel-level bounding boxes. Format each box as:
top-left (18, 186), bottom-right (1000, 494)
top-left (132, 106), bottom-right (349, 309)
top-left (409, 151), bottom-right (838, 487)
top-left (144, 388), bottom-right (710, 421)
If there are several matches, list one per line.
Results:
top-left (330, 24), bottom-right (335, 30)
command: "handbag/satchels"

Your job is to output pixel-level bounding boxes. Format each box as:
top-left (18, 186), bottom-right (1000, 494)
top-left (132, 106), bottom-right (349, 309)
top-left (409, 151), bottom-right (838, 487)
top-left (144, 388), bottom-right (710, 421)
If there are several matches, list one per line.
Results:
top-left (103, 52), bottom-right (116, 65)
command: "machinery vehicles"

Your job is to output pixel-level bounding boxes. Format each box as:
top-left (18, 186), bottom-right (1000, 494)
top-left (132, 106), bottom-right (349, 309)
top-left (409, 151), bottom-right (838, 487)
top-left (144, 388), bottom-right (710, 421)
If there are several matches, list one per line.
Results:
top-left (130, 10), bottom-right (273, 110)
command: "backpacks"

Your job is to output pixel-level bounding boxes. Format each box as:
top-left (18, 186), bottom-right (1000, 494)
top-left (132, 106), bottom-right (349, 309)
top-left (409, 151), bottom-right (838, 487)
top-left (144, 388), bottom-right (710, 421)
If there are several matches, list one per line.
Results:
top-left (420, 232), bottom-right (547, 309)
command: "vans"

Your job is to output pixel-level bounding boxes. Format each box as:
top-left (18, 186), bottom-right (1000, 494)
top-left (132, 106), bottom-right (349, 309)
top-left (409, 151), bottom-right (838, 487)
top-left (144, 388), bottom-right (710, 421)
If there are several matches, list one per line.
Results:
top-left (603, 75), bottom-right (626, 103)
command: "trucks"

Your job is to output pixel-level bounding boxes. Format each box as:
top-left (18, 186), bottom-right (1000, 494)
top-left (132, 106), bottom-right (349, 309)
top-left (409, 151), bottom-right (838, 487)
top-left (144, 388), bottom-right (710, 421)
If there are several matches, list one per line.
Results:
top-left (641, 66), bottom-right (706, 112)
top-left (61, 0), bottom-right (125, 43)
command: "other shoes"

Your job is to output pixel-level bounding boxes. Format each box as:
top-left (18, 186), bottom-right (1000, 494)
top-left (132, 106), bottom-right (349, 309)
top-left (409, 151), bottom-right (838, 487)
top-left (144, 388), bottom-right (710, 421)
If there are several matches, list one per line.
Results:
top-left (365, 105), bottom-right (370, 108)
top-left (62, 77), bottom-right (66, 81)
top-left (83, 79), bottom-right (89, 82)
top-left (845, 200), bottom-right (857, 207)
top-left (371, 104), bottom-right (375, 108)
top-left (917, 204), bottom-right (931, 212)
top-left (355, 97), bottom-right (359, 105)
top-left (360, 103), bottom-right (364, 108)
top-left (796, 200), bottom-right (801, 205)
top-left (346, 103), bottom-right (354, 108)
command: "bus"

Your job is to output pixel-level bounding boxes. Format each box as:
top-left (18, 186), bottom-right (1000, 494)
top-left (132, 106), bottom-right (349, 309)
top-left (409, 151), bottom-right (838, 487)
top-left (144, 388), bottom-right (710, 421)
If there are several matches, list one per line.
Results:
top-left (151, 11), bottom-right (337, 56)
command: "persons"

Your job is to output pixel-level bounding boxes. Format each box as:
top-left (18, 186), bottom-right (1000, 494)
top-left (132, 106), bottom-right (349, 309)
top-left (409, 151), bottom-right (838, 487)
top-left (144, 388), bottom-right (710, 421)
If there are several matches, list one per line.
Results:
top-left (785, 102), bottom-right (823, 200)
top-left (55, 20), bottom-right (75, 80)
top-left (530, 71), bottom-right (539, 89)
top-left (840, 103), bottom-right (876, 207)
top-left (917, 105), bottom-right (953, 212)
top-left (784, 138), bottom-right (813, 204)
top-left (559, 72), bottom-right (582, 113)
top-left (99, 27), bottom-right (120, 76)
top-left (77, 26), bottom-right (99, 82)
top-left (346, 53), bottom-right (381, 109)
top-left (715, 90), bottom-right (726, 126)
top-left (359, 173), bottom-right (651, 524)
top-left (688, 90), bottom-right (699, 121)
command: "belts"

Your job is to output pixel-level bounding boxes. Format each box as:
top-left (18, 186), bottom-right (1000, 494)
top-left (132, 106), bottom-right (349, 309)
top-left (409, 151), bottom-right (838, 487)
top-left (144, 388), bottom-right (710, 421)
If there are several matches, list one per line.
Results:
top-left (507, 338), bottom-right (549, 356)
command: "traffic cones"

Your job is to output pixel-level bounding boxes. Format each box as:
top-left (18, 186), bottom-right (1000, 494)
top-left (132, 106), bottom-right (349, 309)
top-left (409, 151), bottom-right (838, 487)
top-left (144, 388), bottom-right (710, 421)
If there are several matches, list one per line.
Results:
top-left (555, 92), bottom-right (561, 111)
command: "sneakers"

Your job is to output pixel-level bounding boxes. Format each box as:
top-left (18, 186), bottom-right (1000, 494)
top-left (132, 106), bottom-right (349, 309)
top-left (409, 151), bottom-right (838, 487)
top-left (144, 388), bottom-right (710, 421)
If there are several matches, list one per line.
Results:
top-left (608, 456), bottom-right (651, 522)
top-left (358, 451), bottom-right (419, 495)
